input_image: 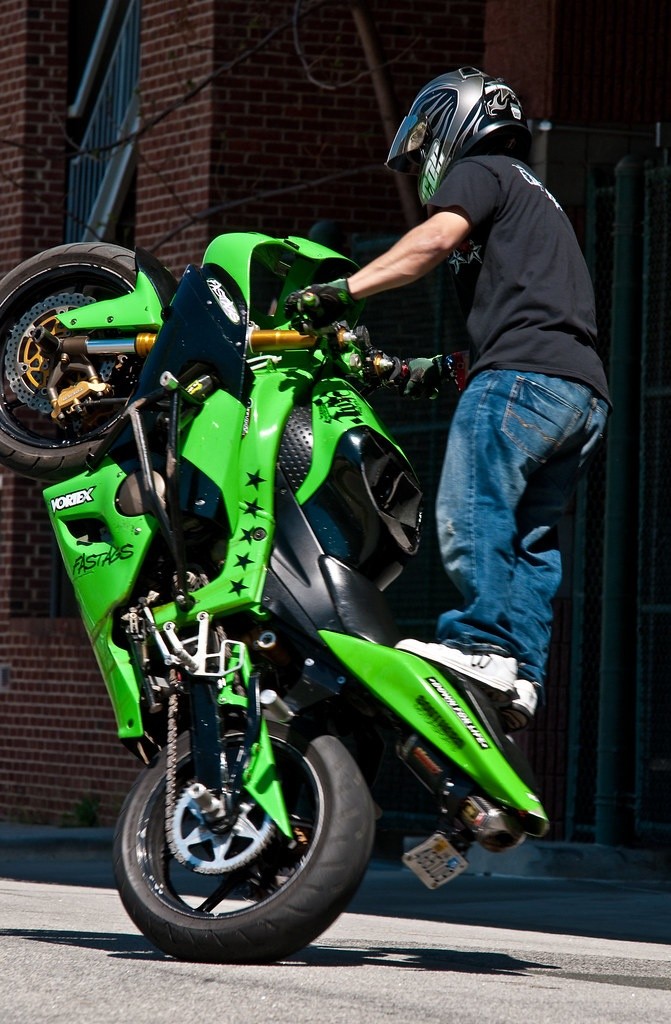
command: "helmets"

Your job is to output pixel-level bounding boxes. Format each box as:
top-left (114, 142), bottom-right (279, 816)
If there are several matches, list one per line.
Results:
top-left (383, 65), bottom-right (533, 206)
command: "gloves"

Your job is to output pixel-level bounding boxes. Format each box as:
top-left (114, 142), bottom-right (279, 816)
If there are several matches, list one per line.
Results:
top-left (281, 277), bottom-right (356, 336)
top-left (403, 354), bottom-right (446, 401)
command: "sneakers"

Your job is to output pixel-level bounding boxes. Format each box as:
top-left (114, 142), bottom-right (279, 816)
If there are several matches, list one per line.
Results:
top-left (488, 678), bottom-right (541, 734)
top-left (395, 638), bottom-right (518, 702)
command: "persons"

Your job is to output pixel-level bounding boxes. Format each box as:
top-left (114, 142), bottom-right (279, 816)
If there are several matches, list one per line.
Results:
top-left (284, 65), bottom-right (612, 733)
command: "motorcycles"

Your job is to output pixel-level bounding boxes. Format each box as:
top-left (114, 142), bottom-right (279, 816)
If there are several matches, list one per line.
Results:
top-left (1, 230), bottom-right (551, 965)
